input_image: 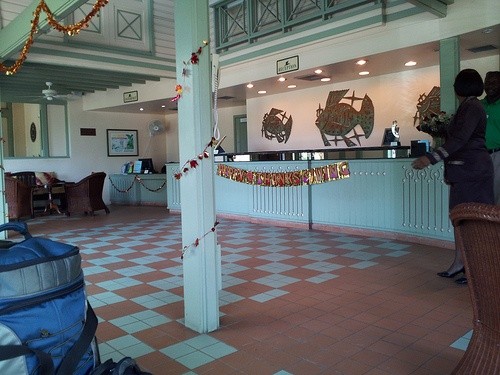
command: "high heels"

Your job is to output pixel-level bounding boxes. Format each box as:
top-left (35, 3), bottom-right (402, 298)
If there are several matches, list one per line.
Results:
top-left (436, 267), bottom-right (466, 279)
top-left (455, 278), bottom-right (468, 285)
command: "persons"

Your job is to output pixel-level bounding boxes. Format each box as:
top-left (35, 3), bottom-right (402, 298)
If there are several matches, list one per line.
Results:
top-left (478, 71), bottom-right (500, 206)
top-left (211, 136), bottom-right (225, 153)
top-left (412, 68), bottom-right (495, 284)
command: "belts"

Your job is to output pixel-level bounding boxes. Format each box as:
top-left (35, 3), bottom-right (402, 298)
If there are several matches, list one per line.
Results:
top-left (488, 148), bottom-right (500, 154)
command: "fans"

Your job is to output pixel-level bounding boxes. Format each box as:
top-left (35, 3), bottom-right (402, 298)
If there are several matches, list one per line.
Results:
top-left (148, 120), bottom-right (163, 137)
top-left (14, 81), bottom-right (81, 101)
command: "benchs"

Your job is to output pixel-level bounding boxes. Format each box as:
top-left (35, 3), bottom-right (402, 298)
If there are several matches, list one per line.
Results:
top-left (12, 172), bottom-right (65, 210)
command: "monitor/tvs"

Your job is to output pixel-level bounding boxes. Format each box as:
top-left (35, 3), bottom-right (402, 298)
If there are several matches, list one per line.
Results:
top-left (138, 158), bottom-right (154, 172)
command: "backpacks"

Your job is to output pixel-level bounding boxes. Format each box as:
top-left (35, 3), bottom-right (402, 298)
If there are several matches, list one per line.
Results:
top-left (0, 222), bottom-right (150, 375)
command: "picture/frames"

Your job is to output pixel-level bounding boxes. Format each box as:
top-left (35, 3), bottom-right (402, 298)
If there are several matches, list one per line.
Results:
top-left (106, 129), bottom-right (139, 156)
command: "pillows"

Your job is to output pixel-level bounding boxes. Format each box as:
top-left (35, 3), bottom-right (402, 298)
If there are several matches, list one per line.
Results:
top-left (35, 172), bottom-right (54, 184)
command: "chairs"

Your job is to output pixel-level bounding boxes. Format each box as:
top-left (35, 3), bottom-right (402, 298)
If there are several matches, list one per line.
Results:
top-left (449, 202), bottom-right (500, 375)
top-left (5, 172), bottom-right (30, 221)
top-left (64, 172), bottom-right (110, 216)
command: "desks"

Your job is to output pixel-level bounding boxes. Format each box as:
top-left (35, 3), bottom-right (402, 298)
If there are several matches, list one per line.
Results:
top-left (33, 183), bottom-right (65, 216)
